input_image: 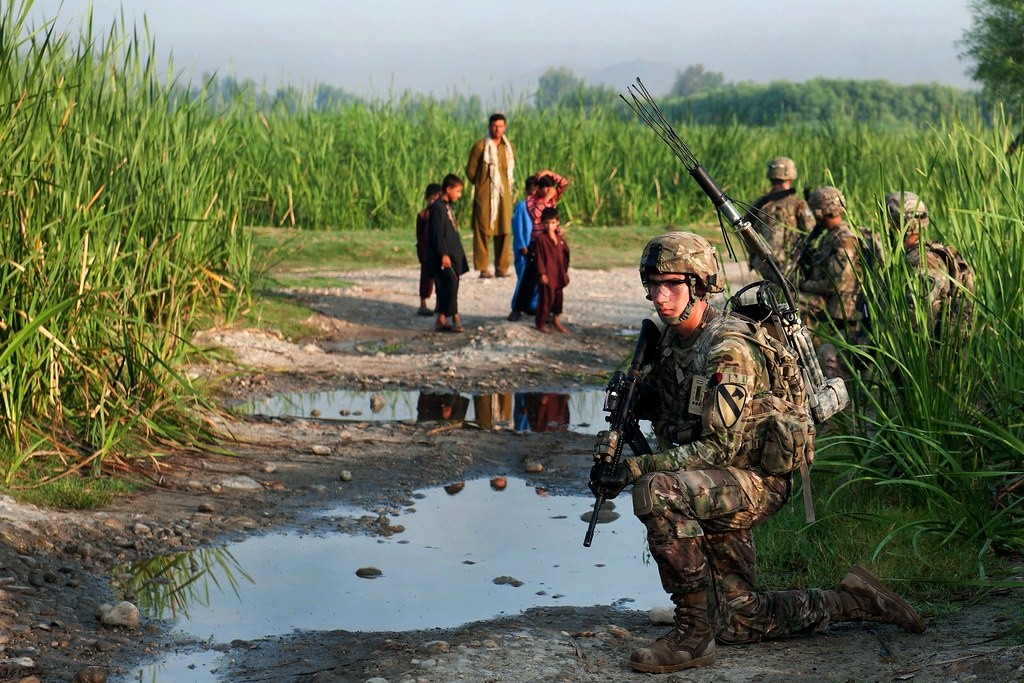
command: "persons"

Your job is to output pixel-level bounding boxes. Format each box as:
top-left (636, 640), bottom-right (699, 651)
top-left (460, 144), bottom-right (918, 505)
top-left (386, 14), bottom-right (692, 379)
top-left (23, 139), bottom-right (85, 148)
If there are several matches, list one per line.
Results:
top-left (743, 155), bottom-right (976, 406)
top-left (588, 232), bottom-right (926, 674)
top-left (415, 170), bottom-right (572, 333)
top-left (465, 113), bottom-right (516, 279)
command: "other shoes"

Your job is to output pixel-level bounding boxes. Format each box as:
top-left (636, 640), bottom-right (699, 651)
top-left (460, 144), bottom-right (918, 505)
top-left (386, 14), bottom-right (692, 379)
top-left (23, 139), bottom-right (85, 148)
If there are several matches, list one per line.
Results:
top-left (453, 319), bottom-right (464, 332)
top-left (435, 322), bottom-right (452, 332)
top-left (479, 271), bottom-right (491, 278)
top-left (497, 273), bottom-right (510, 277)
top-left (417, 306), bottom-right (434, 316)
top-left (508, 311), bottom-right (521, 321)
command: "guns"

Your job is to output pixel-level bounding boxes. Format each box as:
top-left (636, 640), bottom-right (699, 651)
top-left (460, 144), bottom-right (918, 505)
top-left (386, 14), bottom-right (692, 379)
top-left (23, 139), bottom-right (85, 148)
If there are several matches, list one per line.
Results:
top-left (582, 319), bottom-right (663, 548)
top-left (855, 251), bottom-right (873, 321)
top-left (786, 223), bottom-right (825, 283)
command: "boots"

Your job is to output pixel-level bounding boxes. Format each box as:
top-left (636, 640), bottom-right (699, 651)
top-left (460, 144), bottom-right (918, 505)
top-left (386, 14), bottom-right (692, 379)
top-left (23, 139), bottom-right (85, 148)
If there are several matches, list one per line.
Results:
top-left (823, 563), bottom-right (924, 634)
top-left (629, 588), bottom-right (716, 673)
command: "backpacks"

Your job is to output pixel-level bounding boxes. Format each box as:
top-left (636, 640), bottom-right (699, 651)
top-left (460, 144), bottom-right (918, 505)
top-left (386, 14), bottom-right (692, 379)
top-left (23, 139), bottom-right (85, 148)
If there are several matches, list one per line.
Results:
top-left (907, 238), bottom-right (974, 320)
top-left (660, 315), bottom-right (815, 471)
top-left (756, 196), bottom-right (802, 264)
top-left (824, 227), bottom-right (888, 301)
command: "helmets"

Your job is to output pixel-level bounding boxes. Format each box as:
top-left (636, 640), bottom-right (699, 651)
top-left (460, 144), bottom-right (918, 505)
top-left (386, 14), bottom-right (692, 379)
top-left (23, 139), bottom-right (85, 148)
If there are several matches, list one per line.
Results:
top-left (639, 232), bottom-right (724, 301)
top-left (766, 157), bottom-right (798, 180)
top-left (808, 186), bottom-right (846, 217)
top-left (880, 190), bottom-right (929, 236)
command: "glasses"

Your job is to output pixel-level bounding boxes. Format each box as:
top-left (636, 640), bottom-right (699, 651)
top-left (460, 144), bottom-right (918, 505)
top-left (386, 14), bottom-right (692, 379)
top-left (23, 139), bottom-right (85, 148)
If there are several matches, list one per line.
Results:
top-left (641, 278), bottom-right (689, 296)
